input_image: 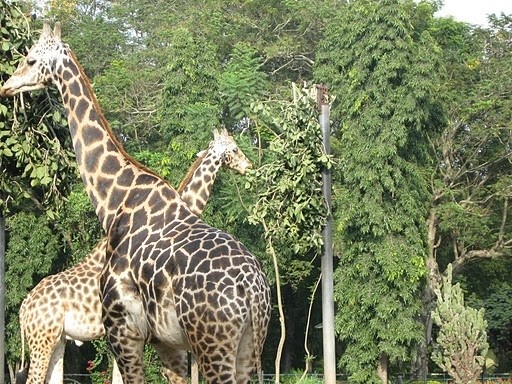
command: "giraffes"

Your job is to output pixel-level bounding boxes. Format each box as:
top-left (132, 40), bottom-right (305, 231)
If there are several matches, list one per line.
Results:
top-left (2, 18), bottom-right (275, 384)
top-left (18, 125), bottom-right (257, 384)
